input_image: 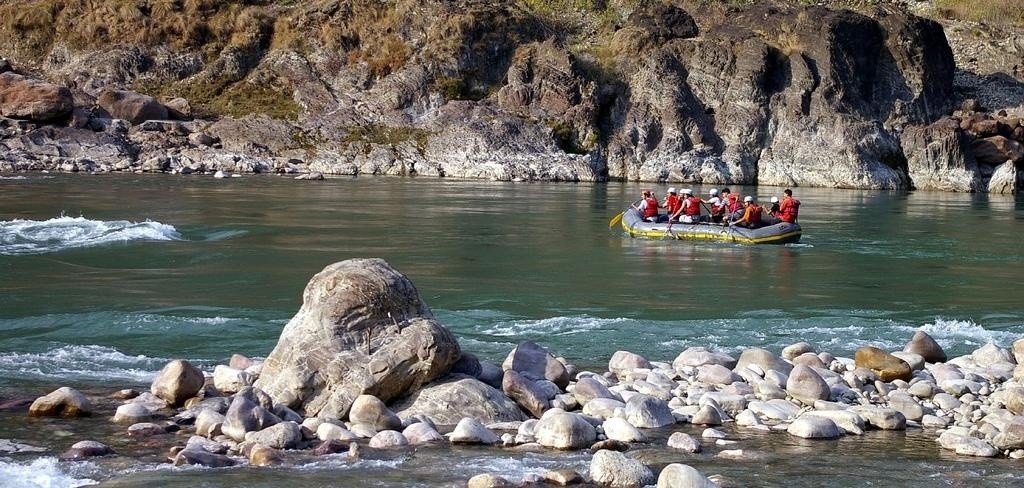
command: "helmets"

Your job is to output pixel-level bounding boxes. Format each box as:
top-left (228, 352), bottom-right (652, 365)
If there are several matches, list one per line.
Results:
top-left (709, 189), bottom-right (718, 196)
top-left (667, 187), bottom-right (692, 196)
top-left (743, 196), bottom-right (753, 202)
top-left (771, 196), bottom-right (779, 203)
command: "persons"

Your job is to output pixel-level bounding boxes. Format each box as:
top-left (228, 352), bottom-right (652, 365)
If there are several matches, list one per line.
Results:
top-left (631, 188), bottom-right (801, 230)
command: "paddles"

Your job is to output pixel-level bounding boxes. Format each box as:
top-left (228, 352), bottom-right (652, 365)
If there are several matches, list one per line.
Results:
top-left (661, 196), bottom-right (681, 236)
top-left (610, 200), bottom-right (642, 227)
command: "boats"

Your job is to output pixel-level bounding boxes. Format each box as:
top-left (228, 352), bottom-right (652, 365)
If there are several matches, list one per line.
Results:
top-left (622, 207), bottom-right (802, 247)
